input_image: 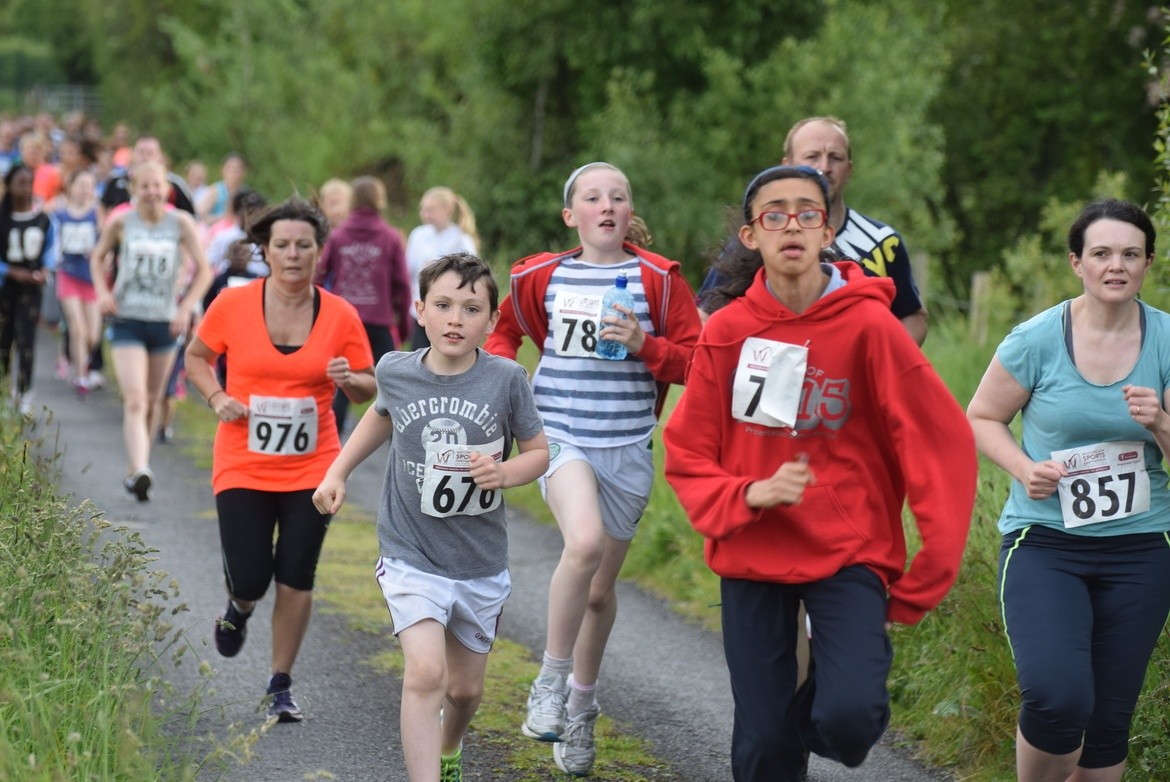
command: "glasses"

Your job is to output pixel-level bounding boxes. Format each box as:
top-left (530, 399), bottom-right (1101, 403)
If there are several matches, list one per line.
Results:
top-left (748, 209), bottom-right (829, 231)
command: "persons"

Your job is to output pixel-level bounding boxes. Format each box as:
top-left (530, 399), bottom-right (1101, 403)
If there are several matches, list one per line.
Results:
top-left (312, 252), bottom-right (550, 782)
top-left (662, 114), bottom-right (979, 782)
top-left (184, 197), bottom-right (378, 724)
top-left (966, 200), bottom-right (1170, 782)
top-left (0, 108), bottom-right (483, 445)
top-left (483, 161), bottom-right (702, 776)
top-left (89, 159), bottom-right (212, 503)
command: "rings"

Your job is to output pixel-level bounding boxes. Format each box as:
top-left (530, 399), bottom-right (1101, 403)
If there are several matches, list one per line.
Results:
top-left (344, 371), bottom-right (348, 378)
top-left (1137, 405), bottom-right (1140, 415)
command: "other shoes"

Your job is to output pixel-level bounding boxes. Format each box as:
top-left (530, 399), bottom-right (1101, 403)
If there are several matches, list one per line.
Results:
top-left (86, 366), bottom-right (104, 391)
top-left (17, 391), bottom-right (34, 416)
top-left (121, 469), bottom-right (153, 502)
top-left (75, 377), bottom-right (89, 396)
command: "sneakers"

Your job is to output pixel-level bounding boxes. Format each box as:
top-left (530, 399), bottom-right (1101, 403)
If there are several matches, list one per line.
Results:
top-left (265, 672), bottom-right (304, 722)
top-left (552, 697), bottom-right (602, 776)
top-left (215, 598), bottom-right (257, 656)
top-left (520, 674), bottom-right (572, 742)
top-left (439, 707), bottom-right (463, 781)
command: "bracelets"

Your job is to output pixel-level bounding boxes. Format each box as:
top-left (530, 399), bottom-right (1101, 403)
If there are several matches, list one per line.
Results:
top-left (207, 389), bottom-right (225, 408)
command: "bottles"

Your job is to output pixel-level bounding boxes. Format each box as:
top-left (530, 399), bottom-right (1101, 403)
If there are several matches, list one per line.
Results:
top-left (597, 273), bottom-right (634, 361)
